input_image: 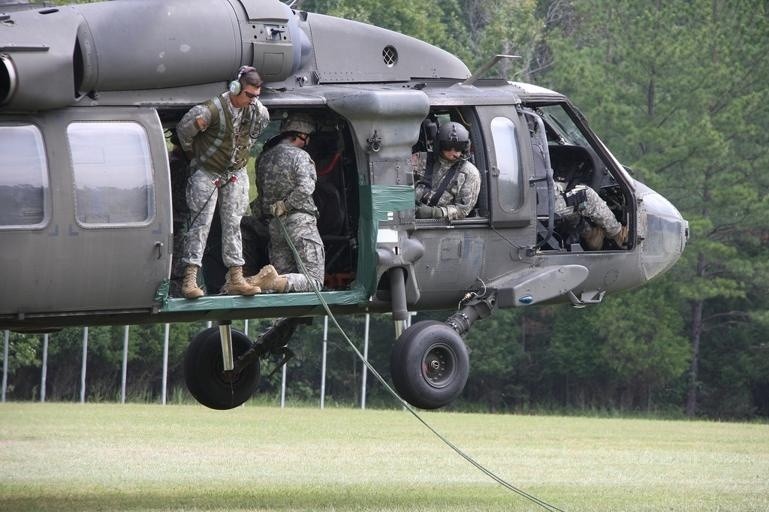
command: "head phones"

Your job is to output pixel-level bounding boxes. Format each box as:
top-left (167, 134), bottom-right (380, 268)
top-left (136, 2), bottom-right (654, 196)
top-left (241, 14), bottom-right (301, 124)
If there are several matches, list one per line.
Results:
top-left (228, 66), bottom-right (257, 96)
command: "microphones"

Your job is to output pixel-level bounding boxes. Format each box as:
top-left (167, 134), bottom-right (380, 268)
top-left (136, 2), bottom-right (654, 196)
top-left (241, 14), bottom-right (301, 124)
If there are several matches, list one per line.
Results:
top-left (454, 155), bottom-right (471, 162)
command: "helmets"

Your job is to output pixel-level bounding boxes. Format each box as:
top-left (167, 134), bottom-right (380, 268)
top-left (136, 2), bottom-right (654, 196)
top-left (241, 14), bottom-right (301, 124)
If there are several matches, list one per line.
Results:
top-left (435, 121), bottom-right (470, 147)
top-left (281, 111), bottom-right (317, 135)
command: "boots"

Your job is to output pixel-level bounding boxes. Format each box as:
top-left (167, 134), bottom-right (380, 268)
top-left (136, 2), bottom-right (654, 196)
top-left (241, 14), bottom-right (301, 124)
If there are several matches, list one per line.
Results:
top-left (582, 223), bottom-right (606, 251)
top-left (612, 226), bottom-right (629, 249)
top-left (245, 264), bottom-right (288, 292)
top-left (227, 267), bottom-right (262, 296)
top-left (181, 266), bottom-right (205, 300)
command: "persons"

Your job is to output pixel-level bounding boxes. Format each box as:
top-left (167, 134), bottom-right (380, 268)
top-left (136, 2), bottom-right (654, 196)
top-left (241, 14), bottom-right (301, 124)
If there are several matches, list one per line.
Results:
top-left (169, 112), bottom-right (326, 293)
top-left (524, 106), bottom-right (631, 253)
top-left (175, 63), bottom-right (271, 299)
top-left (409, 121), bottom-right (481, 222)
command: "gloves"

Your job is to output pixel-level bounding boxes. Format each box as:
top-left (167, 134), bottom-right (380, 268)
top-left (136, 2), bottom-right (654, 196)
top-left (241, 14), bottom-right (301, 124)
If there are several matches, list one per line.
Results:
top-left (270, 200), bottom-right (287, 216)
top-left (415, 199), bottom-right (442, 218)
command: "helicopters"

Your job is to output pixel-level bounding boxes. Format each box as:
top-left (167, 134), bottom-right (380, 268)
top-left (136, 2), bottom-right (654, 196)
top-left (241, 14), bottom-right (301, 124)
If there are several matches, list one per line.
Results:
top-left (0, 0), bottom-right (693, 411)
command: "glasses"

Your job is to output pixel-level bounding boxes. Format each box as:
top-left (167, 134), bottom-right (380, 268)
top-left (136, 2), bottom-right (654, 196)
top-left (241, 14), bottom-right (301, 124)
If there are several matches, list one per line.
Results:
top-left (439, 140), bottom-right (468, 152)
top-left (245, 91), bottom-right (257, 98)
